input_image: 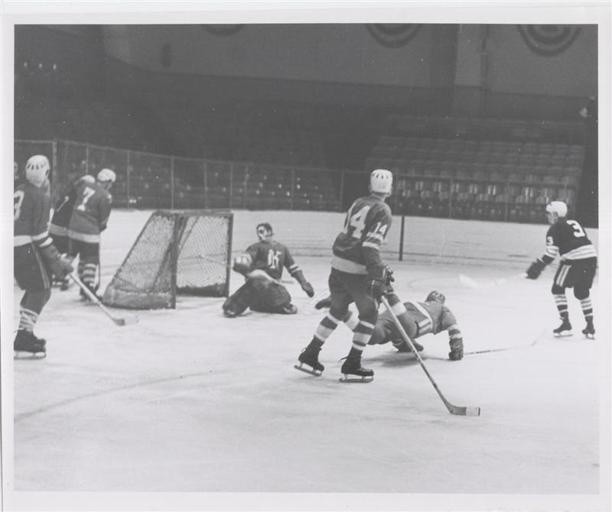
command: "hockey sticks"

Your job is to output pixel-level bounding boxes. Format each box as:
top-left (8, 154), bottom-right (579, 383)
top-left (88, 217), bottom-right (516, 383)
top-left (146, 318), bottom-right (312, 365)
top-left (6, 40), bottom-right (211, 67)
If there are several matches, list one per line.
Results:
top-left (382, 296), bottom-right (480, 416)
top-left (70, 272), bottom-right (141, 327)
top-left (203, 256), bottom-right (294, 287)
top-left (459, 272), bottom-right (529, 289)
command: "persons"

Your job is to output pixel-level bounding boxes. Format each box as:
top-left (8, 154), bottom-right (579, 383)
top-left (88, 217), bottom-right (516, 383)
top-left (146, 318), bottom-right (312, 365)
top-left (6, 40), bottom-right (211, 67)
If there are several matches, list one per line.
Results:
top-left (296, 168), bottom-right (397, 377)
top-left (315, 289), bottom-right (465, 362)
top-left (63, 168), bottom-right (116, 298)
top-left (15, 154), bottom-right (72, 354)
top-left (48, 174), bottom-right (95, 288)
top-left (523, 199), bottom-right (599, 336)
top-left (220, 222), bottom-right (315, 320)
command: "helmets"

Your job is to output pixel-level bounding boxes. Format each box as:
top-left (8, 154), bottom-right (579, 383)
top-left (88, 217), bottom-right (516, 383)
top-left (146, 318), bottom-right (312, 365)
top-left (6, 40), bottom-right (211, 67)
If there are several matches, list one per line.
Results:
top-left (24, 154), bottom-right (52, 188)
top-left (370, 169), bottom-right (394, 194)
top-left (51, 270), bottom-right (105, 307)
top-left (427, 290), bottom-right (445, 304)
top-left (545, 200), bottom-right (569, 219)
top-left (97, 168), bottom-right (117, 184)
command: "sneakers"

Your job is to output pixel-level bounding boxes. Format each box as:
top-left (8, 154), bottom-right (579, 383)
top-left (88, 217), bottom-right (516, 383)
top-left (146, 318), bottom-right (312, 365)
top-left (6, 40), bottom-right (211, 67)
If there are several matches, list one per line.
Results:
top-left (314, 292), bottom-right (333, 309)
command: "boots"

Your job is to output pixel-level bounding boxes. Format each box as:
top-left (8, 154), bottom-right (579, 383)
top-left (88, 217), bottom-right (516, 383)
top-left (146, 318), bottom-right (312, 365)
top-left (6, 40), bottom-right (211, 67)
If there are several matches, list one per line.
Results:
top-left (340, 355), bottom-right (376, 376)
top-left (553, 319), bottom-right (574, 333)
top-left (15, 330), bottom-right (48, 352)
top-left (299, 343), bottom-right (326, 369)
top-left (582, 321), bottom-right (597, 336)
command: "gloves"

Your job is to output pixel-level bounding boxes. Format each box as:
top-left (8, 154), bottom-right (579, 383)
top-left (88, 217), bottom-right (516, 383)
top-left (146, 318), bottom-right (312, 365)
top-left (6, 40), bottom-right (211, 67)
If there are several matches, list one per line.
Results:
top-left (525, 262), bottom-right (544, 280)
top-left (50, 259), bottom-right (75, 282)
top-left (398, 339), bottom-right (424, 354)
top-left (449, 341), bottom-right (465, 360)
top-left (367, 276), bottom-right (389, 303)
